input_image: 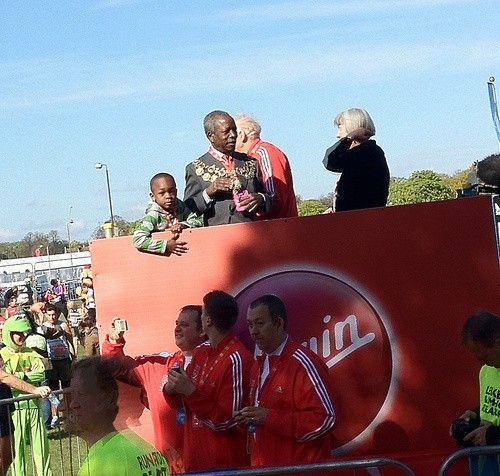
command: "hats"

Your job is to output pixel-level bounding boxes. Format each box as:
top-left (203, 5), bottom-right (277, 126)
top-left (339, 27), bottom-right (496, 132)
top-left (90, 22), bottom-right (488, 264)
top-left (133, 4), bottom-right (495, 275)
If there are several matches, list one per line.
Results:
top-left (39, 245), bottom-right (44, 248)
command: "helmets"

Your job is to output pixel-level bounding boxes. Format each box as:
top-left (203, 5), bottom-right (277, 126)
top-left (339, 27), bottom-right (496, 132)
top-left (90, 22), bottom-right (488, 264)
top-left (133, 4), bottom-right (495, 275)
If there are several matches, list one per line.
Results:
top-left (2, 315), bottom-right (32, 351)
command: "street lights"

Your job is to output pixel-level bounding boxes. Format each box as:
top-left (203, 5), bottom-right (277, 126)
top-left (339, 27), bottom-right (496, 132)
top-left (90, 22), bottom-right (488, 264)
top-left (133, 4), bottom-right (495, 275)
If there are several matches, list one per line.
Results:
top-left (66, 220), bottom-right (74, 280)
top-left (94, 161), bottom-right (115, 238)
top-left (39, 244), bottom-right (52, 284)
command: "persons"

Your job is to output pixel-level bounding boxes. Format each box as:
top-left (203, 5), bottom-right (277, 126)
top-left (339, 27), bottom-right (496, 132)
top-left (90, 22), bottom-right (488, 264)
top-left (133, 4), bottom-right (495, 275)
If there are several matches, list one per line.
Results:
top-left (450, 312), bottom-right (500, 476)
top-left (323, 109), bottom-right (390, 213)
top-left (184, 110), bottom-right (271, 225)
top-left (235, 114), bottom-right (298, 221)
top-left (58, 354), bottom-right (170, 476)
top-left (0, 265), bottom-right (101, 476)
top-left (103, 305), bottom-right (206, 473)
top-left (232, 295), bottom-right (337, 476)
top-left (132, 173), bottom-right (203, 256)
top-left (163, 291), bottom-right (254, 472)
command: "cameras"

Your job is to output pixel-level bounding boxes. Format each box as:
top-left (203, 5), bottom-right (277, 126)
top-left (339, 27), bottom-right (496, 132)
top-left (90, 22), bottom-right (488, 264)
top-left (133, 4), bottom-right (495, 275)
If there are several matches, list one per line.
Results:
top-left (452, 419), bottom-right (482, 451)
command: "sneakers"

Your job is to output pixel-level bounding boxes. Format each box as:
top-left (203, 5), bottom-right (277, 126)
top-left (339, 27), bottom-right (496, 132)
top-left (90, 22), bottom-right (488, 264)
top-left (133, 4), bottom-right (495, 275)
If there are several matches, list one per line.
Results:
top-left (51, 416), bottom-right (58, 428)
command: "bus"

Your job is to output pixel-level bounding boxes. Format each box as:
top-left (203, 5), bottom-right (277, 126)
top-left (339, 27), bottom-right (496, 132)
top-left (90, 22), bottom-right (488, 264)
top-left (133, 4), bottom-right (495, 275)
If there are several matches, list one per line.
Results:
top-left (0, 251), bottom-right (90, 306)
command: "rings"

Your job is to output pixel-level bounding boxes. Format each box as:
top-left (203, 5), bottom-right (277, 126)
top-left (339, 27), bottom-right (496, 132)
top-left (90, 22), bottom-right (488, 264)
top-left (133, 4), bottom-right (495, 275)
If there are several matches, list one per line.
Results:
top-left (252, 417), bottom-right (254, 421)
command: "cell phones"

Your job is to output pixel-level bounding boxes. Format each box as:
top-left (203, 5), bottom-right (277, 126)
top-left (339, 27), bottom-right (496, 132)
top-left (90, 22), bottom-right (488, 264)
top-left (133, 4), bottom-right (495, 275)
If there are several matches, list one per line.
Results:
top-left (115, 318), bottom-right (128, 331)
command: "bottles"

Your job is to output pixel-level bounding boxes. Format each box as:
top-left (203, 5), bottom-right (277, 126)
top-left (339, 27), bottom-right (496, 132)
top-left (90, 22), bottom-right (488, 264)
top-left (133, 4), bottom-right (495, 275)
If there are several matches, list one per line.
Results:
top-left (233, 179), bottom-right (250, 212)
top-left (48, 392), bottom-right (63, 409)
